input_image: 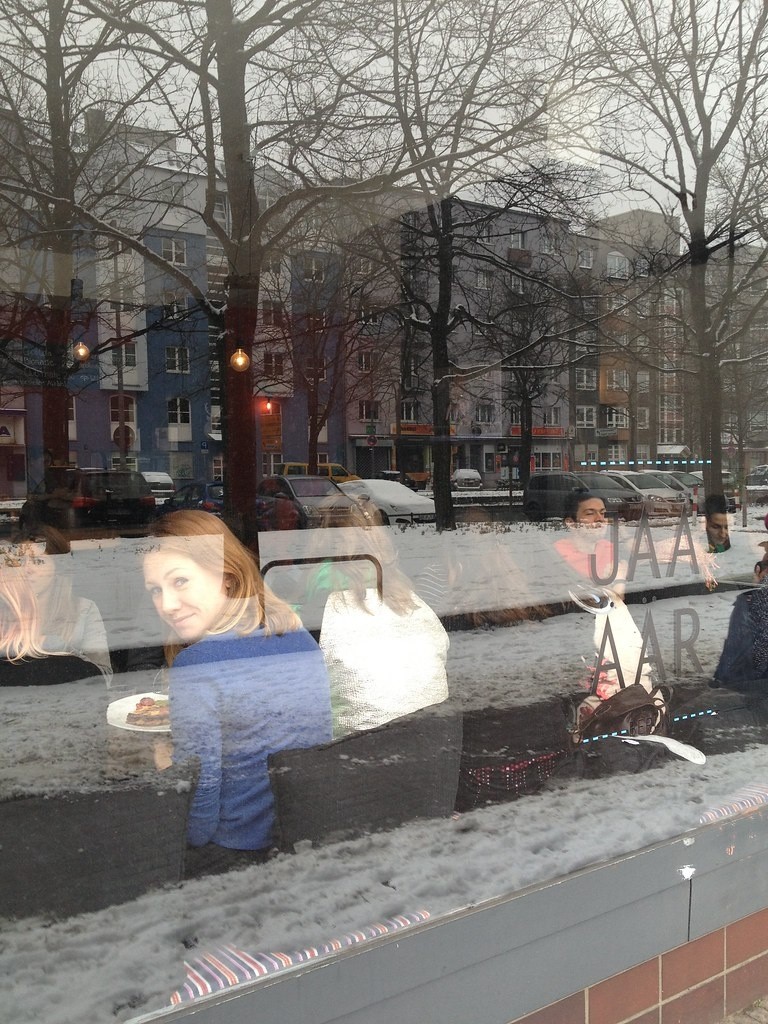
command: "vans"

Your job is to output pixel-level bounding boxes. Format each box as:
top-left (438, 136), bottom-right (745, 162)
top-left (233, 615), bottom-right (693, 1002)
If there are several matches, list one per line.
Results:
top-left (522, 469), bottom-right (737, 521)
top-left (279, 462), bottom-right (362, 489)
top-left (140, 471), bottom-right (176, 506)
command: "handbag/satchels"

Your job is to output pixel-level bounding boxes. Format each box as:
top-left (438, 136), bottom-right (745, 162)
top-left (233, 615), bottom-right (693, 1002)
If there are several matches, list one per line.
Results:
top-left (581, 684), bottom-right (673, 739)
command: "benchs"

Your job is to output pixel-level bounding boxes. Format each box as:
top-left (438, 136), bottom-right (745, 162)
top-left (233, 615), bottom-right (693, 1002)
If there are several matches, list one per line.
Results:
top-left (495, 480), bottom-right (518, 491)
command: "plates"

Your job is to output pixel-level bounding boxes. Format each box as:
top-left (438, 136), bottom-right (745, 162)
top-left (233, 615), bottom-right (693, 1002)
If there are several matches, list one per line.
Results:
top-left (107, 692), bottom-right (171, 732)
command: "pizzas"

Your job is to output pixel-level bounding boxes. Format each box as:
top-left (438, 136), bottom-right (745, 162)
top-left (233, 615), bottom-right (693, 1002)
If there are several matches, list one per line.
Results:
top-left (126, 705), bottom-right (170, 726)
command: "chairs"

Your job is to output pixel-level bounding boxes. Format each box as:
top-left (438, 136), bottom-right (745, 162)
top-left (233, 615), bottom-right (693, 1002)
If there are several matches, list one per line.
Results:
top-left (268, 694), bottom-right (465, 853)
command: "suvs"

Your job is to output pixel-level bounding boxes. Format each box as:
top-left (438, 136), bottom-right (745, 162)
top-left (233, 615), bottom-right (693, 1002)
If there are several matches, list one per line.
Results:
top-left (20, 467), bottom-right (156, 540)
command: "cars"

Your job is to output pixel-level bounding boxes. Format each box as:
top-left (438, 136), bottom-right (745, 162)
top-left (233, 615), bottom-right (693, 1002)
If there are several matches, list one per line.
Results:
top-left (327, 479), bottom-right (436, 525)
top-left (158, 482), bottom-right (276, 531)
top-left (258, 474), bottom-right (362, 527)
top-left (371, 471), bottom-right (419, 493)
top-left (450, 468), bottom-right (484, 491)
top-left (746, 465), bottom-right (768, 503)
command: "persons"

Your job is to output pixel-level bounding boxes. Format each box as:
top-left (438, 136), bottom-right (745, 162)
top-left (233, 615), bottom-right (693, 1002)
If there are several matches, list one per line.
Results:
top-left (421, 489), bottom-right (768, 810)
top-left (0, 494), bottom-right (183, 694)
top-left (303, 494), bottom-right (452, 739)
top-left (141, 509), bottom-right (332, 880)
